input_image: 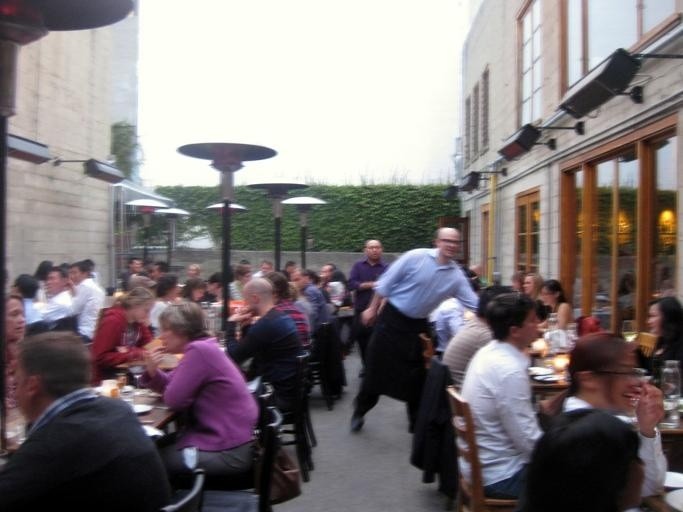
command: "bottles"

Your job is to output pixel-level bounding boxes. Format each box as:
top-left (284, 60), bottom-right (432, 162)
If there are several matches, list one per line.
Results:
top-left (660, 361), bottom-right (681, 429)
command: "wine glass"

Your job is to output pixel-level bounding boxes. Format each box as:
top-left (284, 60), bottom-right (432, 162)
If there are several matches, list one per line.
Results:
top-left (130, 367), bottom-right (143, 393)
top-left (117, 375), bottom-right (126, 398)
top-left (623, 322), bottom-right (639, 346)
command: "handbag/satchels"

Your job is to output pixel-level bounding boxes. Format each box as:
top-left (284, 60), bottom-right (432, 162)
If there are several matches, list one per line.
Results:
top-left (252, 443), bottom-right (301, 505)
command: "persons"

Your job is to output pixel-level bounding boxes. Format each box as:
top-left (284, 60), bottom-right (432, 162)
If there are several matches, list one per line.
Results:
top-left (0, 226), bottom-right (683, 512)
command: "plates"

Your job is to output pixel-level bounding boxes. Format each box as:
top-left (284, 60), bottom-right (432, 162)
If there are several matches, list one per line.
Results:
top-left (664, 490), bottom-right (682, 512)
top-left (664, 471), bottom-right (683, 487)
top-left (529, 366), bottom-right (553, 377)
top-left (537, 375), bottom-right (565, 385)
top-left (131, 403), bottom-right (152, 414)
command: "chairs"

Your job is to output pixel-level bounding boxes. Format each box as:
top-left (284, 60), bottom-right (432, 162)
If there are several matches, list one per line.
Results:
top-left (160, 471), bottom-right (206, 512)
top-left (576, 316), bottom-right (599, 336)
top-left (55, 314), bottom-right (79, 332)
top-left (419, 333), bottom-right (432, 367)
top-left (423, 354), bottom-right (457, 510)
top-left (25, 320), bottom-right (44, 336)
top-left (636, 333), bottom-right (657, 357)
top-left (227, 321), bottom-right (332, 511)
top-left (446, 387), bottom-right (518, 511)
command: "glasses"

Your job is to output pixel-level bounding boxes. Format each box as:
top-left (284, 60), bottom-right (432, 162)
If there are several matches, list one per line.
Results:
top-left (583, 368), bottom-right (645, 382)
top-left (440, 238), bottom-right (460, 246)
top-left (242, 274), bottom-right (251, 278)
top-left (365, 246), bottom-right (380, 251)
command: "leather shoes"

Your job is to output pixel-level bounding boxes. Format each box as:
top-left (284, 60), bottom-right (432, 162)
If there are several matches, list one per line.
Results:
top-left (350, 406), bottom-right (363, 432)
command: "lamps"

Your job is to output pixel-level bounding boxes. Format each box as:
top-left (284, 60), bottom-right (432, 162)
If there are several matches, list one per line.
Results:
top-left (498, 122), bottom-right (584, 161)
top-left (55, 157), bottom-right (124, 183)
top-left (461, 168), bottom-right (506, 190)
top-left (553, 49), bottom-right (683, 122)
top-left (7, 134), bottom-right (49, 164)
top-left (443, 185), bottom-right (471, 201)
